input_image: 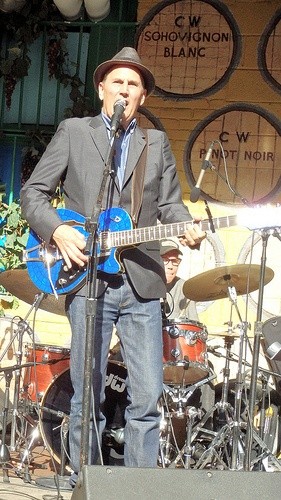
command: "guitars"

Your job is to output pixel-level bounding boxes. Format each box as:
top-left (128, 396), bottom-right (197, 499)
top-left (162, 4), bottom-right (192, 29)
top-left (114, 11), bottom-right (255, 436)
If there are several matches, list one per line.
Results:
top-left (25, 206), bottom-right (244, 295)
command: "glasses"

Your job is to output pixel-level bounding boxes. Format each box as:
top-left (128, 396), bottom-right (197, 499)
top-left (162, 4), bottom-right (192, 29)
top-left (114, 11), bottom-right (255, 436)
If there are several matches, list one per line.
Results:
top-left (162, 257), bottom-right (182, 266)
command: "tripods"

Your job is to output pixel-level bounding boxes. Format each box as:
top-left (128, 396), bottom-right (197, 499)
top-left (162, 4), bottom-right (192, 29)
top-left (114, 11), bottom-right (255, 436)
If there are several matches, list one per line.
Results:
top-left (157, 160), bottom-right (281, 471)
top-left (0, 293), bottom-right (70, 483)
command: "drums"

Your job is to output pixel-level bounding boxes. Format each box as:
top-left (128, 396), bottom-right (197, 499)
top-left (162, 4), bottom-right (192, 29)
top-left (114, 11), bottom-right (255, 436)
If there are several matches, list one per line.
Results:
top-left (160, 318), bottom-right (210, 386)
top-left (195, 377), bottom-right (281, 469)
top-left (0, 317), bottom-right (41, 432)
top-left (38, 359), bottom-right (131, 474)
top-left (22, 342), bottom-right (71, 405)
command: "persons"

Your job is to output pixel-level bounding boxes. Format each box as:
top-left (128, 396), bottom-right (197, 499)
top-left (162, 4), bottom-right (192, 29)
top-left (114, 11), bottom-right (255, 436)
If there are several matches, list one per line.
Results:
top-left (18, 48), bottom-right (206, 491)
top-left (108, 239), bottom-right (215, 447)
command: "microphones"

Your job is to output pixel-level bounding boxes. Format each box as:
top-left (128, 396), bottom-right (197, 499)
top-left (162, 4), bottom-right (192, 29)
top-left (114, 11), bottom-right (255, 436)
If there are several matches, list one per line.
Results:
top-left (163, 298), bottom-right (170, 313)
top-left (19, 317), bottom-right (35, 340)
top-left (110, 99), bottom-right (127, 137)
top-left (189, 142), bottom-right (213, 203)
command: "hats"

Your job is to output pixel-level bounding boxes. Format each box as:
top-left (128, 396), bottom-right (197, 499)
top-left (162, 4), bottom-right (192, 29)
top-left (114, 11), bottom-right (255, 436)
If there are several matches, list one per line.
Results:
top-left (159, 239), bottom-right (184, 256)
top-left (93, 47), bottom-right (155, 99)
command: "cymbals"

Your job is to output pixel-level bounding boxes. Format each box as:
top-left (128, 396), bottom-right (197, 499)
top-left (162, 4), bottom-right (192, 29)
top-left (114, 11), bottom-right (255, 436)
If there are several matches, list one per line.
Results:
top-left (0, 269), bottom-right (69, 316)
top-left (182, 263), bottom-right (275, 302)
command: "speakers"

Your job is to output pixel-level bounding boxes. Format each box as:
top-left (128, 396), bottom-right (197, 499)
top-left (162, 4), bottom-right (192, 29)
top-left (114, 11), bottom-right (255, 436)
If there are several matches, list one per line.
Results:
top-left (70, 465), bottom-right (281, 500)
